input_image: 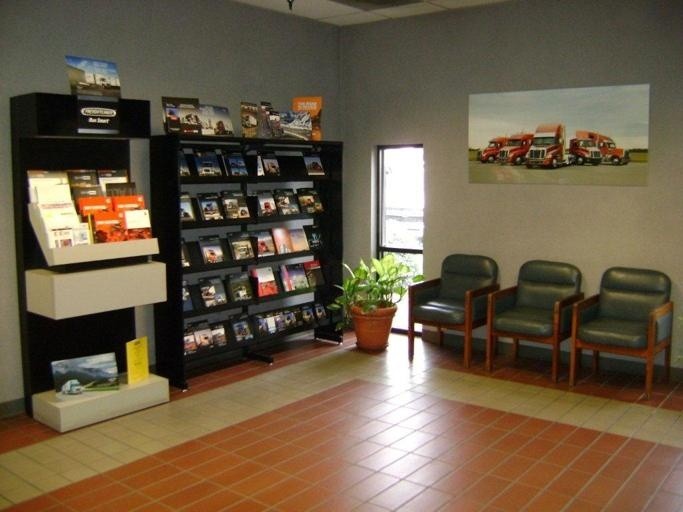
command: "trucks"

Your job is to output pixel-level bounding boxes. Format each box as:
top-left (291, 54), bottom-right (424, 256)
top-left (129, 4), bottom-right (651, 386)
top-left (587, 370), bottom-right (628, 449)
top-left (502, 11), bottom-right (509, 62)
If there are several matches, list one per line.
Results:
top-left (60, 379), bottom-right (83, 395)
top-left (83, 72), bottom-right (111, 89)
top-left (479, 123), bottom-right (629, 170)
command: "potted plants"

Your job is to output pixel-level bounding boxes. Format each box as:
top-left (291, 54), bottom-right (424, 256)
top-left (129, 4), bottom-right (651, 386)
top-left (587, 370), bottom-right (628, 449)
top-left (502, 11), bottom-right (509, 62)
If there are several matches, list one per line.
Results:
top-left (327, 250), bottom-right (424, 352)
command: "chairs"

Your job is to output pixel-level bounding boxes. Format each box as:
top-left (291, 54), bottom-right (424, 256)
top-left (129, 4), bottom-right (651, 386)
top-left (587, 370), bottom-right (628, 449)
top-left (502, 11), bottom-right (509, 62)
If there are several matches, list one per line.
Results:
top-left (406, 253), bottom-right (675, 400)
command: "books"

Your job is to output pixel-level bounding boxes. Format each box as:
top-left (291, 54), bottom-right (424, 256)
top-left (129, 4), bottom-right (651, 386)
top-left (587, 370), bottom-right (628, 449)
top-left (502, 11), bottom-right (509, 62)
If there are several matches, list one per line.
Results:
top-left (175, 150), bottom-right (326, 355)
top-left (162, 95), bottom-right (324, 141)
top-left (26, 164), bottom-right (154, 250)
